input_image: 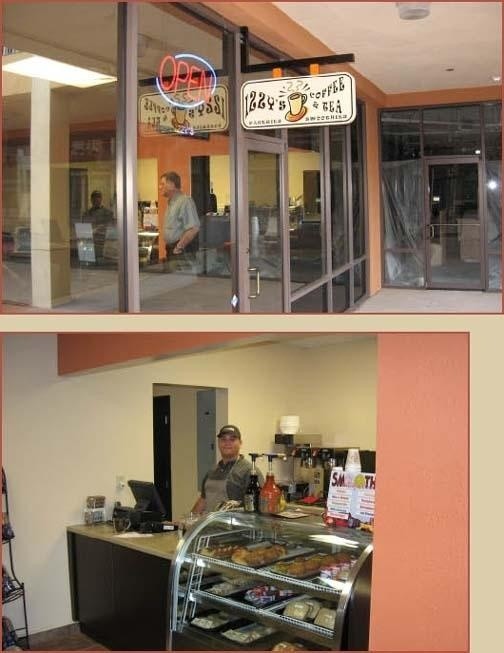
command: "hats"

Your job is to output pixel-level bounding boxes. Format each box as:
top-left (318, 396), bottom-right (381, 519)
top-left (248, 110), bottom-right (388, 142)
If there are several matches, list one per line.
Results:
top-left (92, 190), bottom-right (101, 197)
top-left (218, 426), bottom-right (240, 438)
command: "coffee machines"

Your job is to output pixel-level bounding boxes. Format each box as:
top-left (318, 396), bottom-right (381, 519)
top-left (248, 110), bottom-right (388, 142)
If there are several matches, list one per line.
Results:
top-left (273, 433), bottom-right (321, 486)
top-left (287, 445), bottom-right (361, 501)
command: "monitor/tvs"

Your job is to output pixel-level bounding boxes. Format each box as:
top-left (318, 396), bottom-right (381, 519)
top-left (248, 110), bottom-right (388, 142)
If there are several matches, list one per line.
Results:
top-left (127, 479), bottom-right (167, 520)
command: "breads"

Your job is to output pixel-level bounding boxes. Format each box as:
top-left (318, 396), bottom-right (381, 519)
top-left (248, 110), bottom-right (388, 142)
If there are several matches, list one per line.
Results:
top-left (201, 542), bottom-right (242, 560)
top-left (232, 544), bottom-right (287, 569)
top-left (284, 599), bottom-right (336, 631)
top-left (276, 549), bottom-right (350, 573)
top-left (274, 643), bottom-right (306, 653)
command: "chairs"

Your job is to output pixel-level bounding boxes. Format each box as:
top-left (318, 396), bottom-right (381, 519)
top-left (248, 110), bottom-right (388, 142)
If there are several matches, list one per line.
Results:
top-left (5, 199), bottom-right (308, 277)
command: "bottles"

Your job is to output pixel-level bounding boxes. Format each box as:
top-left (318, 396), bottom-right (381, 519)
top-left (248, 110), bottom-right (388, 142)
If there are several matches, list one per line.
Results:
top-left (148, 198), bottom-right (156, 214)
top-left (243, 468), bottom-right (281, 513)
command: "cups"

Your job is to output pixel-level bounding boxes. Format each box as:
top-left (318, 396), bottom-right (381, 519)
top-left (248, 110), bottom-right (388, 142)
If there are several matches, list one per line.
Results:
top-left (345, 447), bottom-right (362, 475)
top-left (110, 514), bottom-right (131, 533)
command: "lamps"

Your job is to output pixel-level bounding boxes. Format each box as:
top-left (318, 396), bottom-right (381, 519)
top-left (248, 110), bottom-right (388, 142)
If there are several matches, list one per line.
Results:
top-left (395, 1), bottom-right (432, 21)
top-left (1, 49), bottom-right (117, 89)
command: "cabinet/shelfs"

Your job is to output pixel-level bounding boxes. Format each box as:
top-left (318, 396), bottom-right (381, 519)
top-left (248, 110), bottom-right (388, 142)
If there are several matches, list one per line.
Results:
top-left (1, 469), bottom-right (37, 652)
top-left (65, 520), bottom-right (186, 651)
top-left (164, 502), bottom-right (372, 653)
top-left (454, 216), bottom-right (481, 263)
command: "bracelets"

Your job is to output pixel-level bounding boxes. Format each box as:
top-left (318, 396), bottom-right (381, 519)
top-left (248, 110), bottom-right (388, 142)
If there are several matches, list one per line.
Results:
top-left (176, 244), bottom-right (185, 251)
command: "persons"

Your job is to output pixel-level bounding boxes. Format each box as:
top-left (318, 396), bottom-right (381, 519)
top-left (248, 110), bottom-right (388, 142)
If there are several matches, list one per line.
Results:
top-left (160, 172), bottom-right (201, 266)
top-left (191, 426), bottom-right (264, 536)
top-left (81, 191), bottom-right (113, 225)
